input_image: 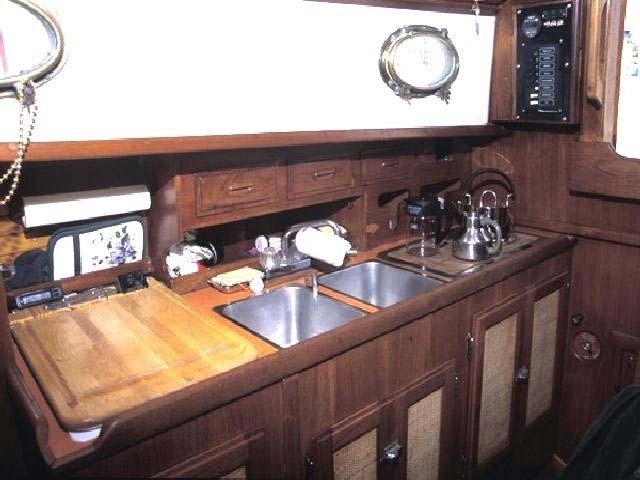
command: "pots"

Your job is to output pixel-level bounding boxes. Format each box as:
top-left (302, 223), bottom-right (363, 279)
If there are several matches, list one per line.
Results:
top-left (458, 190), bottom-right (515, 243)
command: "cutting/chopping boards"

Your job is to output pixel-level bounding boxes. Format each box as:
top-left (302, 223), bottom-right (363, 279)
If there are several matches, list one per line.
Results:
top-left (8, 274), bottom-right (259, 435)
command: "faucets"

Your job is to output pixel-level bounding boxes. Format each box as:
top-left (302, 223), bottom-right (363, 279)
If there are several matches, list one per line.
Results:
top-left (279, 220), bottom-right (348, 271)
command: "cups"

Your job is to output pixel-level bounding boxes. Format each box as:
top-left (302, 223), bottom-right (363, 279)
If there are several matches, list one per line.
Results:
top-left (403, 196), bottom-right (453, 257)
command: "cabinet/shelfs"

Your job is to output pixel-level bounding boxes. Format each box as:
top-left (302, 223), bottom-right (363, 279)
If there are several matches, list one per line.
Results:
top-left (304, 357), bottom-right (462, 479)
top-left (139, 139), bottom-right (472, 295)
top-left (461, 269), bottom-right (572, 477)
top-left (146, 428), bottom-right (287, 480)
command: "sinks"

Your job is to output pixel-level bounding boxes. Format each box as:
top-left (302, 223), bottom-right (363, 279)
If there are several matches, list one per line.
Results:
top-left (315, 258), bottom-right (456, 310)
top-left (212, 282), bottom-right (373, 352)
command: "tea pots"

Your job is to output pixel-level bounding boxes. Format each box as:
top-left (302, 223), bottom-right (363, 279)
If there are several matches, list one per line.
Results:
top-left (453, 210), bottom-right (504, 261)
